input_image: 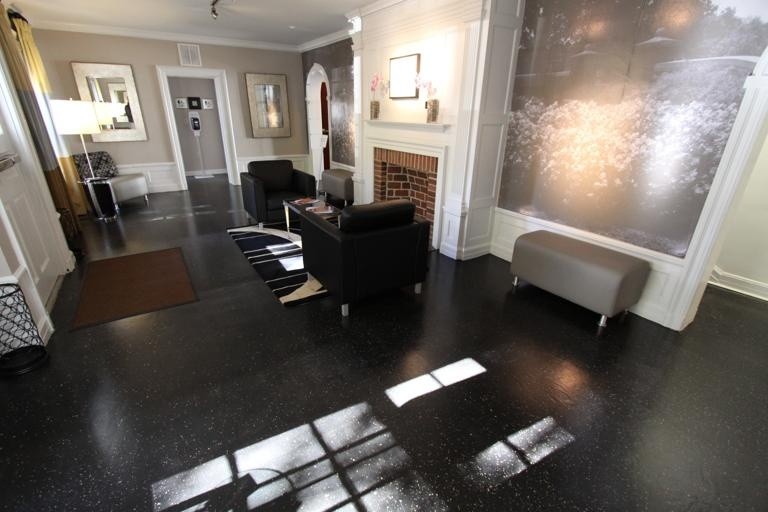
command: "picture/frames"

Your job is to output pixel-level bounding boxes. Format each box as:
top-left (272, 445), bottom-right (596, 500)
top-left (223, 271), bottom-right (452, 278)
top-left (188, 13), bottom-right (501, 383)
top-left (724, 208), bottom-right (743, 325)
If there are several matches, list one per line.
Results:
top-left (388, 53), bottom-right (421, 99)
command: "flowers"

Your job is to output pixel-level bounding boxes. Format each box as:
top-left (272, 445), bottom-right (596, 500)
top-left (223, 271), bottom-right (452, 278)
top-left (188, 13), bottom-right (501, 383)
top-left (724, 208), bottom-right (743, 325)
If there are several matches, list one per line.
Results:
top-left (369, 71), bottom-right (383, 100)
top-left (416, 73), bottom-right (436, 99)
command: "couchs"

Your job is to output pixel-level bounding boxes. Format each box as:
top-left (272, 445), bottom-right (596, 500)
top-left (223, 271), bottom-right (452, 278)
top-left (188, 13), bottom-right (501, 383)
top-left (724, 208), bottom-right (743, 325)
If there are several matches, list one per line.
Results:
top-left (299, 199), bottom-right (430, 317)
top-left (321, 168), bottom-right (353, 207)
top-left (509, 229), bottom-right (651, 328)
top-left (240, 160), bottom-right (315, 230)
top-left (72, 151), bottom-right (149, 210)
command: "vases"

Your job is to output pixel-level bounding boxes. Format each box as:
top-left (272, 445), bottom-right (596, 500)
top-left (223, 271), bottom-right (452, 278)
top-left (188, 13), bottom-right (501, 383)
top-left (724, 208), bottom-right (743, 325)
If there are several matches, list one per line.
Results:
top-left (370, 101), bottom-right (380, 120)
top-left (426, 99), bottom-right (439, 123)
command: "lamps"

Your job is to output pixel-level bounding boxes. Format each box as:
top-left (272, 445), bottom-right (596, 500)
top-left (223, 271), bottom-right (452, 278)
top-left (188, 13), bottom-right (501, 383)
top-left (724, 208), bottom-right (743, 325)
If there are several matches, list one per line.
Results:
top-left (48, 97), bottom-right (117, 221)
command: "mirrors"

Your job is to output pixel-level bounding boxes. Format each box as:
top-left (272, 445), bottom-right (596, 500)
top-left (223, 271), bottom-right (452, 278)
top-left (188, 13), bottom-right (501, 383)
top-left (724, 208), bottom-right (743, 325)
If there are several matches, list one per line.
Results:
top-left (70, 62), bottom-right (148, 142)
top-left (245, 72), bottom-right (291, 138)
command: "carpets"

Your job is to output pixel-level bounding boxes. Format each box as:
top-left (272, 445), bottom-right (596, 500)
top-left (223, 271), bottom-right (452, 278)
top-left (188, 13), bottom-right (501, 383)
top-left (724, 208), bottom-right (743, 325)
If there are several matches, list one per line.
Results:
top-left (226, 219), bottom-right (330, 307)
top-left (68, 246), bottom-right (200, 332)
